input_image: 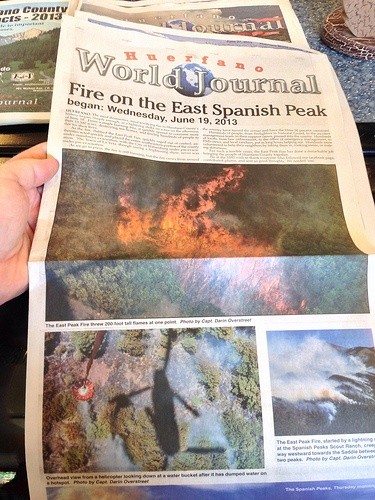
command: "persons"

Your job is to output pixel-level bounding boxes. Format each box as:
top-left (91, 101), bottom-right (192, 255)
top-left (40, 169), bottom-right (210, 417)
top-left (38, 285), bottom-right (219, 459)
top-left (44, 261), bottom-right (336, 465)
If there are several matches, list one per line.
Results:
top-left (0, 140), bottom-right (60, 306)
